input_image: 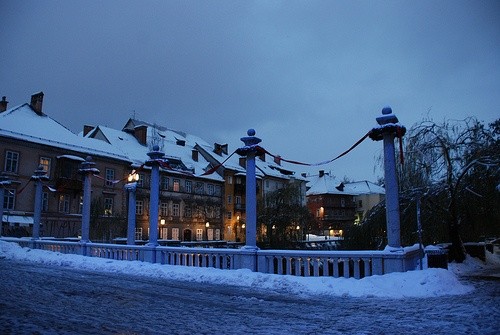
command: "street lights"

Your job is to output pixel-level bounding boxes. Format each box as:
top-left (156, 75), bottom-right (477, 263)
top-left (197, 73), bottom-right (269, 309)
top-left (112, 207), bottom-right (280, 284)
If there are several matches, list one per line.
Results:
top-left (160, 218), bottom-right (165, 239)
top-left (205, 221), bottom-right (210, 241)
